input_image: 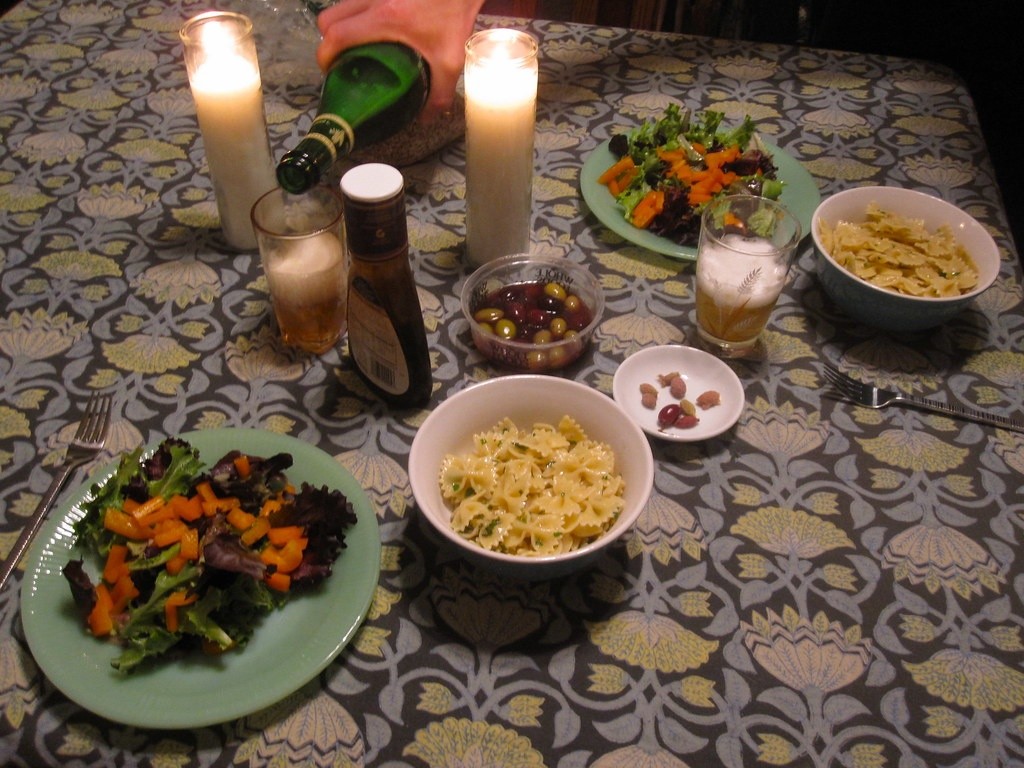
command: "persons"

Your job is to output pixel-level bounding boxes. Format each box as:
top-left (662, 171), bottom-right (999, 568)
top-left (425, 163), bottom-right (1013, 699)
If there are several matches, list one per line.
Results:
top-left (318, 1), bottom-right (485, 123)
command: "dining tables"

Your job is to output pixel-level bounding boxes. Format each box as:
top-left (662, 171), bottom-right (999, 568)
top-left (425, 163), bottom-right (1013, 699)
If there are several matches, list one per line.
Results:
top-left (0, 0), bottom-right (1024, 768)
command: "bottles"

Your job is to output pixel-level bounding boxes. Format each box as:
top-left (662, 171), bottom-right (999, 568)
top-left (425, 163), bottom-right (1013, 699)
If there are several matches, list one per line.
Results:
top-left (276, 40), bottom-right (431, 195)
top-left (340, 163), bottom-right (433, 413)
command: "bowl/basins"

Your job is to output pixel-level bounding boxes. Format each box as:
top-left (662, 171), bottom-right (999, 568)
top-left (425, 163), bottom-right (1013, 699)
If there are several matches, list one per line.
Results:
top-left (810, 186), bottom-right (1001, 333)
top-left (461, 253), bottom-right (605, 372)
top-left (409, 375), bottom-right (654, 563)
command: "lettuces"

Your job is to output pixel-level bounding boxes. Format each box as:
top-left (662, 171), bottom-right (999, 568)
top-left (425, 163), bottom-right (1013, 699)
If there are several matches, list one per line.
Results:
top-left (64, 435), bottom-right (358, 672)
top-left (608, 100), bottom-right (784, 246)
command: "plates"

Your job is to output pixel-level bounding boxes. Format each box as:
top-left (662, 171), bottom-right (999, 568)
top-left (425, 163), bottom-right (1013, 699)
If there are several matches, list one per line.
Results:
top-left (613, 345), bottom-right (745, 442)
top-left (581, 122), bottom-right (821, 261)
top-left (20, 428), bottom-right (381, 729)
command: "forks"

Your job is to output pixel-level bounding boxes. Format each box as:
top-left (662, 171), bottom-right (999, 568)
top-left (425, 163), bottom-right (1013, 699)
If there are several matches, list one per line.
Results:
top-left (0, 391), bottom-right (112, 589)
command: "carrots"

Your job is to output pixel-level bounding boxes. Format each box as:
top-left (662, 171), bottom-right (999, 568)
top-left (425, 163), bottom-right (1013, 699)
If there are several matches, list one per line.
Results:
top-left (598, 142), bottom-right (763, 228)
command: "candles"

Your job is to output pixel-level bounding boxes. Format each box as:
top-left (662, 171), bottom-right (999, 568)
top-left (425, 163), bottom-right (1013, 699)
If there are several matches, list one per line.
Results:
top-left (178, 10), bottom-right (286, 250)
top-left (463, 27), bottom-right (539, 272)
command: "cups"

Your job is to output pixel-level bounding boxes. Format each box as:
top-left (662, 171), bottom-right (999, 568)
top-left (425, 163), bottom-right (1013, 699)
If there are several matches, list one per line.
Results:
top-left (694, 195), bottom-right (802, 359)
top-left (250, 186), bottom-right (348, 354)
